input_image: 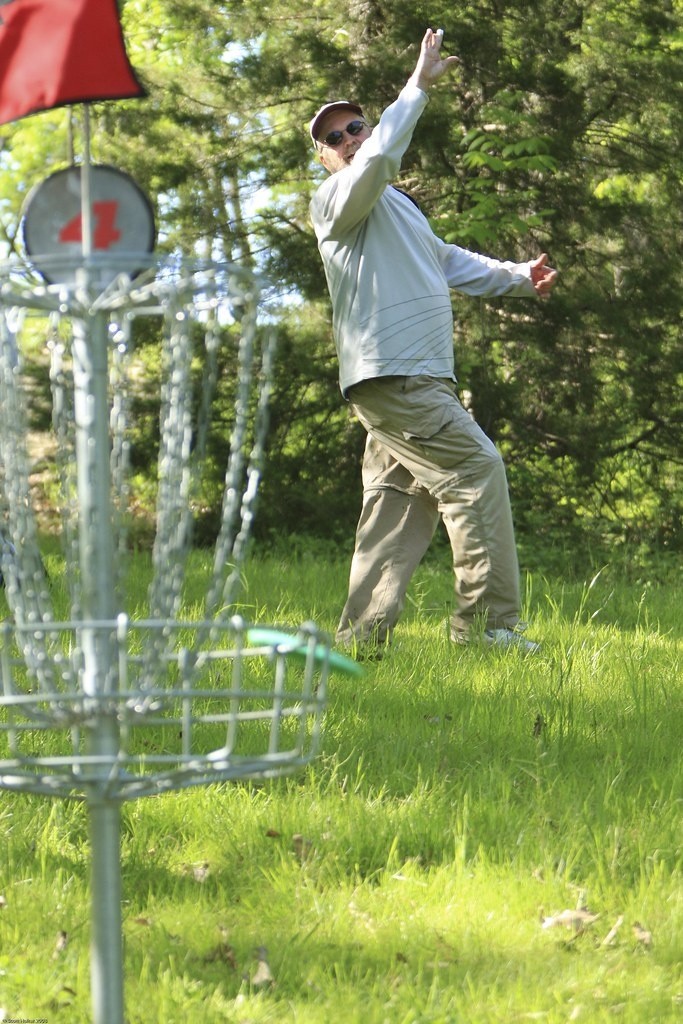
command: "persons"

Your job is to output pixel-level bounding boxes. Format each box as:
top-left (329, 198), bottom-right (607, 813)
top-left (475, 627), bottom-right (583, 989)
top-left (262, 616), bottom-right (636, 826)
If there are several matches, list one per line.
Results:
top-left (309, 28), bottom-right (558, 662)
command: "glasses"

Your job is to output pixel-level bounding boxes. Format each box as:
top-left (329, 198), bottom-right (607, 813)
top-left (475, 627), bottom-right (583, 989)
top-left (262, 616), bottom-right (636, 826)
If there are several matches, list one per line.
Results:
top-left (321, 121), bottom-right (369, 154)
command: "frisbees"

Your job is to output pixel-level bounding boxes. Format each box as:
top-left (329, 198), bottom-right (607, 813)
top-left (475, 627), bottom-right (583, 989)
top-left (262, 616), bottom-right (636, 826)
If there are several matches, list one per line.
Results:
top-left (246, 627), bottom-right (368, 678)
top-left (21, 164), bottom-right (158, 285)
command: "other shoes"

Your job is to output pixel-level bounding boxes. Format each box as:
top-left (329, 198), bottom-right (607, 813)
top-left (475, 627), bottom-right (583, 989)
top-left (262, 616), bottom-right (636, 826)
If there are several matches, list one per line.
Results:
top-left (450, 625), bottom-right (540, 653)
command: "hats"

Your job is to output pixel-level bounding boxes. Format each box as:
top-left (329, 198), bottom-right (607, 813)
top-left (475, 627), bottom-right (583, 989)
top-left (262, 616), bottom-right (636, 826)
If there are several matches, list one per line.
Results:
top-left (310, 101), bottom-right (363, 148)
